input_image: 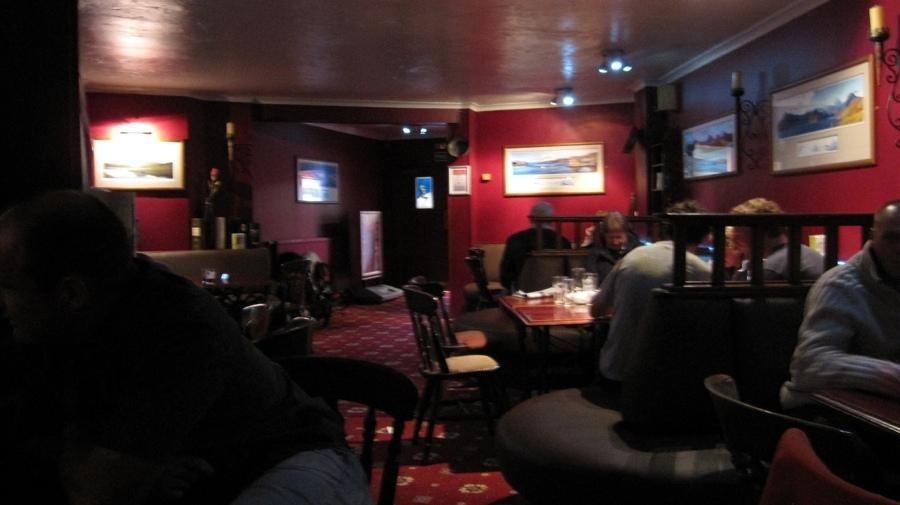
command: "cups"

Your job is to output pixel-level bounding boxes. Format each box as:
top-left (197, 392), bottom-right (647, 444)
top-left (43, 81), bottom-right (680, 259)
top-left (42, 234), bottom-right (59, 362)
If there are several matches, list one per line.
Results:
top-left (201, 266), bottom-right (217, 285)
top-left (809, 234), bottom-right (825, 255)
top-left (551, 266), bottom-right (601, 306)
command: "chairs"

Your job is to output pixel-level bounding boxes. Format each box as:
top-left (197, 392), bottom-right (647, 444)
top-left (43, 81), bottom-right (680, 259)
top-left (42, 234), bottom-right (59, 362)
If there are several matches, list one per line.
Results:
top-left (402, 271), bottom-right (504, 457)
top-left (465, 239), bottom-right (874, 499)
top-left (273, 261), bottom-right (312, 340)
top-left (284, 355), bottom-right (419, 501)
top-left (317, 259), bottom-right (339, 325)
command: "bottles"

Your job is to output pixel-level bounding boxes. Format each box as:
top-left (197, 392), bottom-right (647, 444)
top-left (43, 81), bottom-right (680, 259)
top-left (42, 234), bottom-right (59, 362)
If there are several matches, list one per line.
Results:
top-left (191, 216), bottom-right (260, 250)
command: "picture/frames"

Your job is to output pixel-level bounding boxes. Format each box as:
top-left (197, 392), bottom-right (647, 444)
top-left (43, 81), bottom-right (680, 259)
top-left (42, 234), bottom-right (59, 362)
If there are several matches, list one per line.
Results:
top-left (678, 108), bottom-right (742, 186)
top-left (294, 154), bottom-right (341, 206)
top-left (88, 135), bottom-right (188, 192)
top-left (764, 52), bottom-right (881, 180)
top-left (448, 162), bottom-right (474, 198)
top-left (500, 141), bottom-right (610, 198)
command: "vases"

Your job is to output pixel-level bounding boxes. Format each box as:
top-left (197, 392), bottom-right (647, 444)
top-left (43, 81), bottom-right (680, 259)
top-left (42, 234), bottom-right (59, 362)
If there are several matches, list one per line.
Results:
top-left (204, 167), bottom-right (221, 200)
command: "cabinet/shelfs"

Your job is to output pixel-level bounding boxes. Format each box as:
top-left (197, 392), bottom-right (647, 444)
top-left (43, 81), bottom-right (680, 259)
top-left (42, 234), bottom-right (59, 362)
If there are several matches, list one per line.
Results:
top-left (644, 124), bottom-right (689, 220)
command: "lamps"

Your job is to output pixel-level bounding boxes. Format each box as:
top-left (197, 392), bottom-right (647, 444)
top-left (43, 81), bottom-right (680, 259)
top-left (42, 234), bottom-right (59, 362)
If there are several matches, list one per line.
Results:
top-left (548, 84), bottom-right (577, 107)
top-left (597, 50), bottom-right (633, 79)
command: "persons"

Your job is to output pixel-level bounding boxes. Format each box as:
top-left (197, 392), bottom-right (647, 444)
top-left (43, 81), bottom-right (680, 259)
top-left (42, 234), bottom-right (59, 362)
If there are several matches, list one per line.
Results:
top-left (579, 208), bottom-right (644, 290)
top-left (772, 198), bottom-right (900, 501)
top-left (724, 196), bottom-right (823, 281)
top-left (1, 188), bottom-right (374, 504)
top-left (570, 199), bottom-right (711, 411)
top-left (497, 201), bottom-right (577, 295)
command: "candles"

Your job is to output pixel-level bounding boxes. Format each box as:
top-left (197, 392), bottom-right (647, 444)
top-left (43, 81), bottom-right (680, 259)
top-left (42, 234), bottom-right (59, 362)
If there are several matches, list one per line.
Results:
top-left (730, 67), bottom-right (746, 90)
top-left (867, 2), bottom-right (886, 38)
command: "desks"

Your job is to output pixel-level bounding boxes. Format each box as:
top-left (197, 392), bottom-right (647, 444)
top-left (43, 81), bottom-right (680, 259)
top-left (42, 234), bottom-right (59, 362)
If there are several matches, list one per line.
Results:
top-left (203, 272), bottom-right (276, 353)
